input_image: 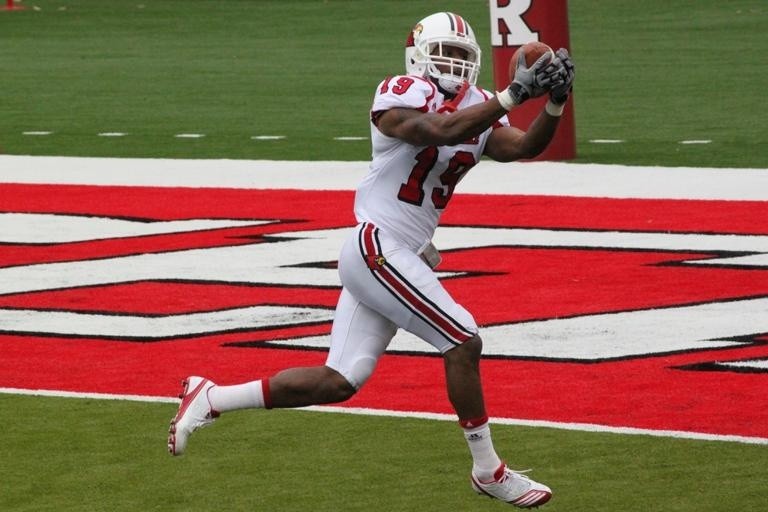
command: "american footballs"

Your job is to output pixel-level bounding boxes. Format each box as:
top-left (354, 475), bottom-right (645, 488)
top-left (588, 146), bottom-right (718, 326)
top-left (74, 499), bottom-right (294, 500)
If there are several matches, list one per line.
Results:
top-left (509, 41), bottom-right (556, 99)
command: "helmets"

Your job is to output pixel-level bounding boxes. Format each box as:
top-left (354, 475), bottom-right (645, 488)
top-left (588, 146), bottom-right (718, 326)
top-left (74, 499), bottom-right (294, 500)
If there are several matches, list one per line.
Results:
top-left (405, 11), bottom-right (482, 95)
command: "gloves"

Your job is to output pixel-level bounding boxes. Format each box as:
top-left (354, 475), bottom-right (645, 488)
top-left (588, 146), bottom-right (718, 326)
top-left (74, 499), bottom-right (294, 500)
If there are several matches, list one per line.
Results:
top-left (495, 47), bottom-right (568, 113)
top-left (543, 48), bottom-right (577, 118)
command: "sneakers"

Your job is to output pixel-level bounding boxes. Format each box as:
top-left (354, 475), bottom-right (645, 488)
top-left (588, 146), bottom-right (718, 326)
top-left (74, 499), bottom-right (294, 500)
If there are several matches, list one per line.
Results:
top-left (167, 375), bottom-right (221, 457)
top-left (470, 461), bottom-right (552, 510)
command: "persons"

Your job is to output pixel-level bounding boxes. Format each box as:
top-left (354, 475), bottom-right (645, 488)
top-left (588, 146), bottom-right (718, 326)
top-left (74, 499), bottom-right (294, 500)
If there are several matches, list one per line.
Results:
top-left (167, 11), bottom-right (573, 509)
